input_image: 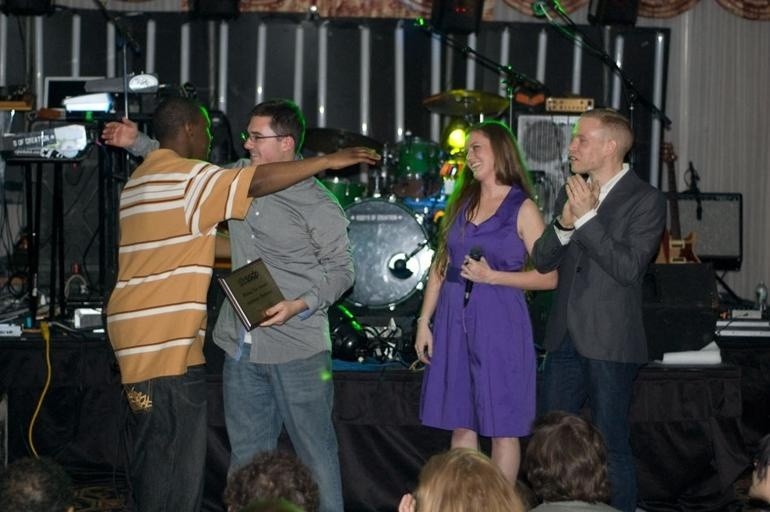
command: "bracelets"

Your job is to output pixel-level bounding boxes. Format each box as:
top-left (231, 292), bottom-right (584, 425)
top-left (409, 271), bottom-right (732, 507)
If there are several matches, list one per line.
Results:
top-left (552, 215), bottom-right (575, 231)
top-left (417, 317), bottom-right (431, 324)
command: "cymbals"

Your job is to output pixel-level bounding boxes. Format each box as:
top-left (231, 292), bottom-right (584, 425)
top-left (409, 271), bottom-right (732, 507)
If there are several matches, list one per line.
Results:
top-left (306, 125), bottom-right (383, 179)
top-left (428, 90), bottom-right (505, 117)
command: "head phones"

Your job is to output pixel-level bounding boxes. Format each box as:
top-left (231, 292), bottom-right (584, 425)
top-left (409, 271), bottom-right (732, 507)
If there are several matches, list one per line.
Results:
top-left (331, 314), bottom-right (367, 361)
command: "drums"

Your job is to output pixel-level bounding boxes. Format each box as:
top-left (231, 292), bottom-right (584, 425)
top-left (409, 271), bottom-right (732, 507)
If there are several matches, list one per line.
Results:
top-left (391, 136), bottom-right (444, 175)
top-left (319, 174), bottom-right (363, 215)
top-left (385, 179), bottom-right (444, 201)
top-left (342, 200), bottom-right (433, 312)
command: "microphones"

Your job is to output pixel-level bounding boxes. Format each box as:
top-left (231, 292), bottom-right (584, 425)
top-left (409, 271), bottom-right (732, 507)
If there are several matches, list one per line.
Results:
top-left (463, 246), bottom-right (483, 308)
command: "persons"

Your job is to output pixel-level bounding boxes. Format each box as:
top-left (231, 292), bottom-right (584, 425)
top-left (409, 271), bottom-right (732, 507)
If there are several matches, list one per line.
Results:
top-left (521, 410), bottom-right (621, 512)
top-left (748, 434), bottom-right (770, 512)
top-left (223, 451), bottom-right (320, 512)
top-left (398, 447), bottom-right (525, 512)
top-left (530, 107), bottom-right (667, 512)
top-left (1, 456), bottom-right (75, 511)
top-left (414, 123), bottom-right (560, 486)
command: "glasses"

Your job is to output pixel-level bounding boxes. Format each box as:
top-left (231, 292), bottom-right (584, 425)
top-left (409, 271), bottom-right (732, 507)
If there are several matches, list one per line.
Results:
top-left (239, 129), bottom-right (285, 144)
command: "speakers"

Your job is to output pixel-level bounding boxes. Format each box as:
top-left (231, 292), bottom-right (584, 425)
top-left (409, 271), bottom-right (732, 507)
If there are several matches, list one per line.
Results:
top-left (587, 0), bottom-right (640, 26)
top-left (641, 262), bottom-right (722, 359)
top-left (431, 1), bottom-right (485, 36)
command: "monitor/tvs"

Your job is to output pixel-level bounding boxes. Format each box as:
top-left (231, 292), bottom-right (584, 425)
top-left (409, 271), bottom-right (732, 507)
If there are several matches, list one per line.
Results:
top-left (44, 76), bottom-right (107, 109)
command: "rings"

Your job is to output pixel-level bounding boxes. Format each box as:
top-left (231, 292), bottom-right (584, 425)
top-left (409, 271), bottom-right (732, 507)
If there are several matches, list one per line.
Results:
top-left (417, 351), bottom-right (424, 357)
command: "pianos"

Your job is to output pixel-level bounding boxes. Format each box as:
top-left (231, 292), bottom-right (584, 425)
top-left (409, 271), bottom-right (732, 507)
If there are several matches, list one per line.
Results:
top-left (62, 93), bottom-right (170, 114)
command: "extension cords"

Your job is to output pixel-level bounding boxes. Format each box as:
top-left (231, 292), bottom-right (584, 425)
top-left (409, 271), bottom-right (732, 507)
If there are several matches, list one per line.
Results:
top-left (40, 320), bottom-right (51, 339)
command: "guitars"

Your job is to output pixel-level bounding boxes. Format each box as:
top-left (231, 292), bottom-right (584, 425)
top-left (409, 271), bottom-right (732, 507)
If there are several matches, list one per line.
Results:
top-left (652, 142), bottom-right (702, 263)
